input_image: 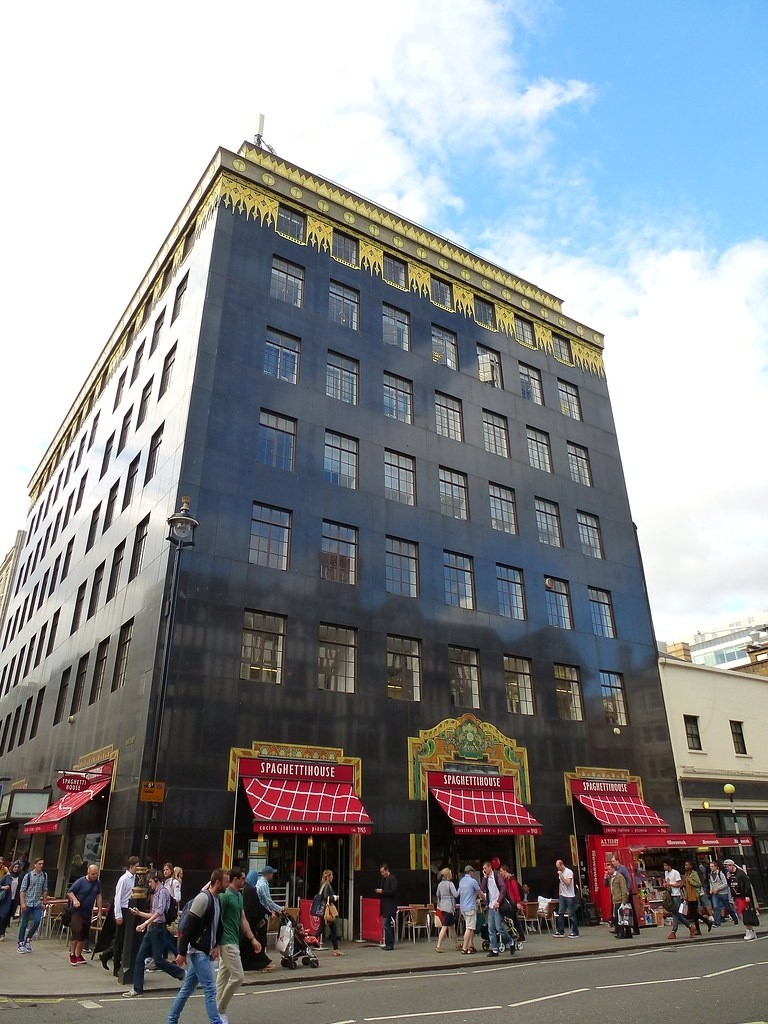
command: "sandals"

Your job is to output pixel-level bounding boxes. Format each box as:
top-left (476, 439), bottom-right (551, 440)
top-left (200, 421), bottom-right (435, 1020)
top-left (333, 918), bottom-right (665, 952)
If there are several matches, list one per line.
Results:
top-left (469, 946), bottom-right (478, 952)
top-left (461, 949), bottom-right (475, 954)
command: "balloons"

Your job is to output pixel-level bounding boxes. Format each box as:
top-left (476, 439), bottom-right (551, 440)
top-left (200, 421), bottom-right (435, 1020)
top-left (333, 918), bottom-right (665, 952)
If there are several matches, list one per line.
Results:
top-left (491, 857), bottom-right (501, 871)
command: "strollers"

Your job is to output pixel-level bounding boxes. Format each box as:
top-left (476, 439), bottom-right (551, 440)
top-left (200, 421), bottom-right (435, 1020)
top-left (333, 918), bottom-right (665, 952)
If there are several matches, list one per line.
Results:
top-left (481, 894), bottom-right (525, 956)
top-left (279, 909), bottom-right (320, 969)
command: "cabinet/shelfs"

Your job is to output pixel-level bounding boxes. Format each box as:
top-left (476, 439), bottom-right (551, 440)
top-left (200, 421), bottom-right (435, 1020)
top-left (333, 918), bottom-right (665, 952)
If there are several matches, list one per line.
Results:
top-left (637, 877), bottom-right (673, 927)
top-left (639, 847), bottom-right (677, 877)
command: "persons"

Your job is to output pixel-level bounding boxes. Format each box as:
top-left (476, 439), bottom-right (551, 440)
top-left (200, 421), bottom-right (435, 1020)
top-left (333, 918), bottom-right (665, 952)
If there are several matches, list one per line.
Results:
top-left (603, 854), bottom-right (760, 942)
top-left (1, 846), bottom-right (309, 968)
top-left (120, 871), bottom-right (188, 998)
top-left (434, 863), bottom-right (532, 957)
top-left (167, 869), bottom-right (230, 1024)
top-left (216, 869), bottom-right (263, 1024)
top-left (314, 869), bottom-right (347, 957)
top-left (552, 860), bottom-right (580, 938)
top-left (376, 866), bottom-right (398, 951)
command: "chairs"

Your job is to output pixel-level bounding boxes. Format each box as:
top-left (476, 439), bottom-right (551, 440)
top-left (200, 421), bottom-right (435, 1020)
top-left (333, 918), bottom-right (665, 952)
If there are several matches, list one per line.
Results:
top-left (264, 908), bottom-right (300, 956)
top-left (520, 896), bottom-right (571, 936)
top-left (388, 902), bottom-right (465, 944)
top-left (40, 893), bottom-right (109, 955)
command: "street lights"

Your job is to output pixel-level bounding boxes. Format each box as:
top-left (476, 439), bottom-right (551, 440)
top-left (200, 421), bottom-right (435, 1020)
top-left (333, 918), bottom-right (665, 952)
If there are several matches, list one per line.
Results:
top-left (724, 783), bottom-right (761, 916)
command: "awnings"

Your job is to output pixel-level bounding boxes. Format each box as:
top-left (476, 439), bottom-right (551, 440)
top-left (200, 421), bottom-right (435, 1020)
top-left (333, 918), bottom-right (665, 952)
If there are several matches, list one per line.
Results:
top-left (22, 778), bottom-right (111, 835)
top-left (427, 786), bottom-right (543, 839)
top-left (577, 794), bottom-right (672, 835)
top-left (243, 776), bottom-right (375, 836)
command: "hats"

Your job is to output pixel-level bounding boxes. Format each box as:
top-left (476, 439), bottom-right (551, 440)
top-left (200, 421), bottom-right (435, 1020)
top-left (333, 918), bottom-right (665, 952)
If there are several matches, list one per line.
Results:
top-left (262, 866), bottom-right (278, 876)
top-left (722, 859), bottom-right (735, 865)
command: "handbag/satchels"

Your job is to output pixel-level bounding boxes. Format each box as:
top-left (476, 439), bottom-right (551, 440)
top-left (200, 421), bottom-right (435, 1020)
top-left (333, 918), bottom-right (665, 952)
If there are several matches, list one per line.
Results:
top-left (500, 896), bottom-right (518, 916)
top-left (62, 906), bottom-right (74, 926)
top-left (617, 903), bottom-right (633, 926)
top-left (280, 923), bottom-right (295, 957)
top-left (434, 909), bottom-right (445, 928)
top-left (678, 901), bottom-right (689, 916)
top-left (743, 900), bottom-right (760, 926)
top-left (324, 896), bottom-right (339, 926)
top-left (310, 883), bottom-right (326, 917)
top-left (0, 889), bottom-right (8, 904)
top-left (275, 921), bottom-right (292, 952)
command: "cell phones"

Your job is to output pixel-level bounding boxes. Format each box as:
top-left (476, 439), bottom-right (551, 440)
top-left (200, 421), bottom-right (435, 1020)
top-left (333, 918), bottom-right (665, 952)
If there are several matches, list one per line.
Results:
top-left (180, 963), bottom-right (187, 970)
top-left (129, 907), bottom-right (136, 913)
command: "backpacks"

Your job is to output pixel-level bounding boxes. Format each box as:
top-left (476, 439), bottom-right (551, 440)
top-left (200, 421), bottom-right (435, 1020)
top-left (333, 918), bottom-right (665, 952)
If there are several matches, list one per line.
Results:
top-left (662, 884), bottom-right (676, 912)
top-left (178, 890), bottom-right (211, 943)
top-left (159, 887), bottom-right (178, 926)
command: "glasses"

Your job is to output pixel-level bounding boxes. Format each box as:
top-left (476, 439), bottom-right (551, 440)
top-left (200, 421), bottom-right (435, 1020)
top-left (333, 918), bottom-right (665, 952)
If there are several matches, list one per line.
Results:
top-left (238, 877), bottom-right (246, 880)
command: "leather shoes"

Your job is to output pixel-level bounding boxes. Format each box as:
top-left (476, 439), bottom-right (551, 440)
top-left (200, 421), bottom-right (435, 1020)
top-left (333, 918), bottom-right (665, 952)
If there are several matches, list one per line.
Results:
top-left (689, 925), bottom-right (696, 937)
top-left (114, 968), bottom-right (121, 976)
top-left (632, 930), bottom-right (641, 935)
top-left (382, 946), bottom-right (394, 950)
top-left (99, 952), bottom-right (110, 970)
top-left (609, 930), bottom-right (617, 933)
top-left (668, 933), bottom-right (677, 939)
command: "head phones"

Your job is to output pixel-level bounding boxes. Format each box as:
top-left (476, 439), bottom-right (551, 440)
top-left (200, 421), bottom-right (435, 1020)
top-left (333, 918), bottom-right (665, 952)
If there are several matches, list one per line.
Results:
top-left (154, 870), bottom-right (159, 883)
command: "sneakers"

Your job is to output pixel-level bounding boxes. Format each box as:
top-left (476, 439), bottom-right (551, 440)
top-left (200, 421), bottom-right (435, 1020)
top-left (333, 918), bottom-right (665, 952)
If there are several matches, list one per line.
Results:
top-left (712, 923), bottom-right (721, 928)
top-left (17, 940), bottom-right (25, 954)
top-left (76, 954), bottom-right (87, 963)
top-left (708, 920), bottom-right (713, 933)
top-left (745, 929), bottom-right (751, 937)
top-left (720, 916), bottom-right (726, 923)
top-left (694, 930), bottom-right (701, 935)
top-left (708, 915), bottom-right (715, 923)
top-left (744, 930), bottom-right (758, 939)
top-left (487, 951), bottom-right (499, 957)
top-left (24, 938), bottom-right (33, 952)
top-left (216, 1007), bottom-right (229, 1024)
top-left (698, 919), bottom-right (704, 923)
top-left (123, 989), bottom-right (143, 997)
top-left (553, 932), bottom-right (565, 937)
top-left (510, 941), bottom-right (516, 955)
top-left (69, 951), bottom-right (78, 966)
top-left (734, 919), bottom-right (739, 926)
top-left (516, 937), bottom-right (527, 943)
top-left (568, 932), bottom-right (580, 938)
top-left (146, 964), bottom-right (163, 972)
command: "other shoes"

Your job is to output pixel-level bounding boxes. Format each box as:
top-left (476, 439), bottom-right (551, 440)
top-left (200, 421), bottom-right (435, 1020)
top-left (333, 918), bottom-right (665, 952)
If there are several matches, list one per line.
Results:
top-left (260, 965), bottom-right (276, 970)
top-left (624, 933), bottom-right (633, 938)
top-left (614, 934), bottom-right (624, 939)
top-left (456, 944), bottom-right (463, 950)
top-left (0, 934), bottom-right (5, 942)
top-left (435, 947), bottom-right (446, 952)
top-left (334, 950), bottom-right (347, 956)
